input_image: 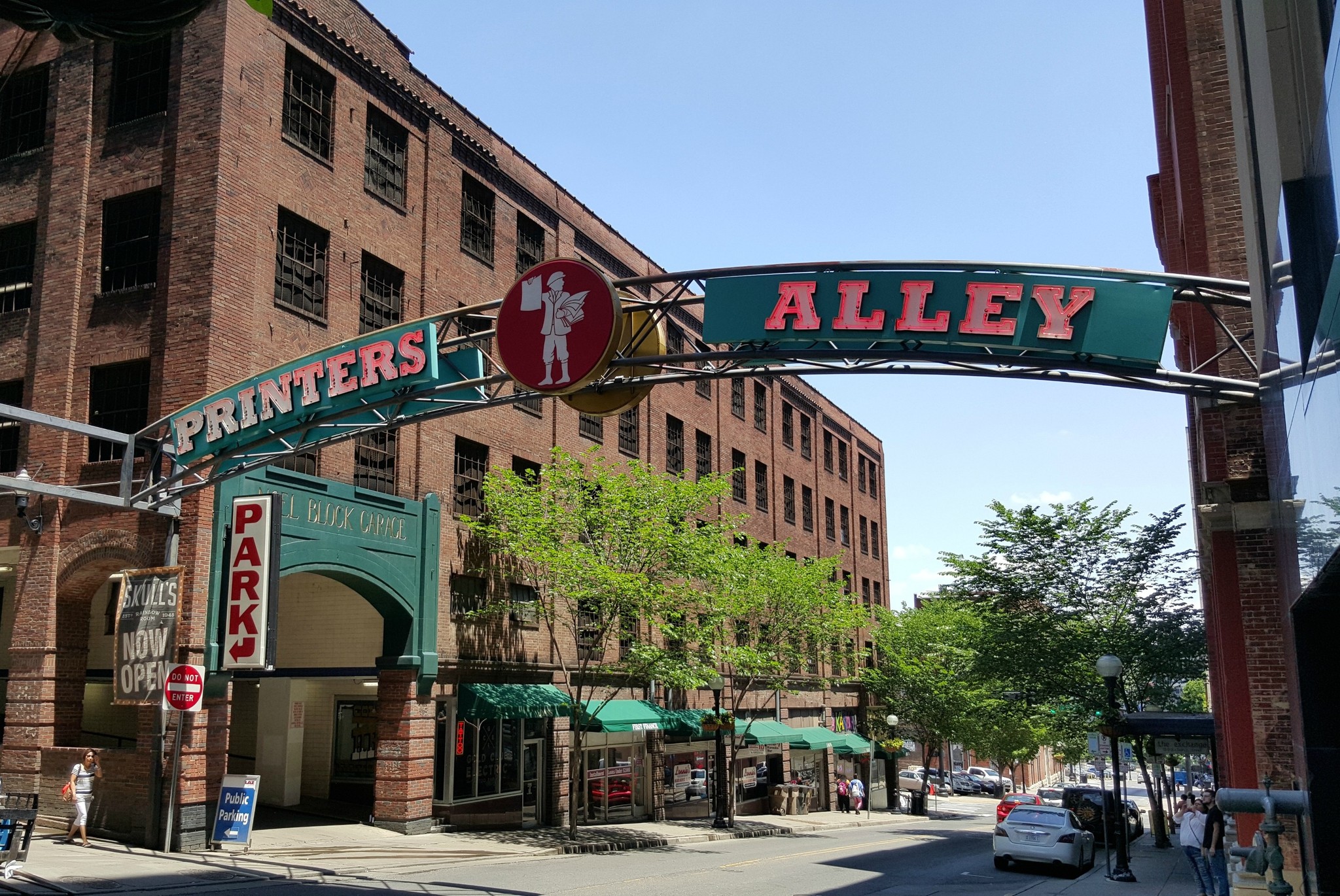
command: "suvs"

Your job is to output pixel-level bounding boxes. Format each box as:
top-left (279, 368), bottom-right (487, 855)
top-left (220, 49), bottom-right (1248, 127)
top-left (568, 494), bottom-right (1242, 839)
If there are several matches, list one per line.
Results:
top-left (1060, 787), bottom-right (1125, 850)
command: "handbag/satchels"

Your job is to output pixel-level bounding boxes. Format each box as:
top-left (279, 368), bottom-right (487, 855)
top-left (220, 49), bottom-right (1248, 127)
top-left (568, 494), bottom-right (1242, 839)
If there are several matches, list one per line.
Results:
top-left (62, 781), bottom-right (71, 794)
top-left (63, 786), bottom-right (72, 803)
top-left (1200, 843), bottom-right (1208, 857)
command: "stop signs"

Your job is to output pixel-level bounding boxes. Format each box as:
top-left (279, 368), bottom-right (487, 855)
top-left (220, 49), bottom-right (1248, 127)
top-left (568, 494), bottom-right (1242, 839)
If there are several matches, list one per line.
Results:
top-left (164, 665), bottom-right (204, 711)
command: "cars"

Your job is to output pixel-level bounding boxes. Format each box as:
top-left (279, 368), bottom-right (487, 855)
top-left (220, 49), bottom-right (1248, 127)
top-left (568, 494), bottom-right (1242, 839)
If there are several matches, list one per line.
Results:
top-left (957, 773), bottom-right (995, 794)
top-left (967, 766), bottom-right (1013, 793)
top-left (1051, 782), bottom-right (1074, 791)
top-left (1114, 798), bottom-right (1146, 840)
top-left (1105, 762), bottom-right (1112, 768)
top-left (1113, 772), bottom-right (1127, 781)
top-left (995, 793), bottom-right (1048, 825)
top-left (1077, 783), bottom-right (1101, 792)
top-left (1075, 768), bottom-right (1096, 779)
top-left (1137, 772), bottom-right (1154, 784)
top-left (951, 773), bottom-right (981, 794)
top-left (1194, 777), bottom-right (1214, 788)
top-left (899, 771), bottom-right (951, 795)
top-left (1087, 767), bottom-right (1113, 778)
top-left (1127, 763), bottom-right (1137, 772)
top-left (1035, 787), bottom-right (1063, 809)
top-left (990, 803), bottom-right (1095, 875)
top-left (1064, 765), bottom-right (1075, 777)
top-left (913, 767), bottom-right (971, 795)
top-left (1161, 774), bottom-right (1172, 783)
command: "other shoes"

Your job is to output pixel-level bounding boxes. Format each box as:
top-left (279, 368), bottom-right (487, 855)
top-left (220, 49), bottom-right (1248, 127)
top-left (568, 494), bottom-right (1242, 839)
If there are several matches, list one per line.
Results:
top-left (846, 811), bottom-right (851, 813)
top-left (855, 810), bottom-right (858, 814)
top-left (842, 811), bottom-right (844, 813)
top-left (856, 807), bottom-right (860, 814)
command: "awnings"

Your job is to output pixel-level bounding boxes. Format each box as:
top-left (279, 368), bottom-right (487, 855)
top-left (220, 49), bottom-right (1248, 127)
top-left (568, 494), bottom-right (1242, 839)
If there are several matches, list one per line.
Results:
top-left (455, 681), bottom-right (585, 720)
top-left (575, 698), bottom-right (683, 734)
top-left (837, 733), bottom-right (883, 755)
top-left (743, 719), bottom-right (803, 746)
top-left (671, 707), bottom-right (752, 736)
top-left (876, 740), bottom-right (910, 760)
top-left (791, 726), bottom-right (848, 751)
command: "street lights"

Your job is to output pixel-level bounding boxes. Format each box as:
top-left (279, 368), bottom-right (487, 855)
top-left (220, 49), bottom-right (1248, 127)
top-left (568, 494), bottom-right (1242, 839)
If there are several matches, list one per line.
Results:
top-left (880, 704), bottom-right (905, 815)
top-left (1098, 647), bottom-right (1135, 881)
top-left (702, 673), bottom-right (735, 829)
top-left (1146, 704), bottom-right (1172, 848)
top-left (1057, 741), bottom-right (1063, 783)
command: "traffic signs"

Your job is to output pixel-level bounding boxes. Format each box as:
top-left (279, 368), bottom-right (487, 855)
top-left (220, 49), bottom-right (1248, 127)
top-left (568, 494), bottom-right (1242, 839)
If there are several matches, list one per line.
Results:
top-left (1116, 741), bottom-right (1133, 864)
top-left (1087, 731), bottom-right (1114, 879)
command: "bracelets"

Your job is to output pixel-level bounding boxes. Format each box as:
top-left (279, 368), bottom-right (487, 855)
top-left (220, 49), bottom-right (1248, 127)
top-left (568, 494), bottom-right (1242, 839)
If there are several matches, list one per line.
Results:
top-left (97, 767), bottom-right (101, 770)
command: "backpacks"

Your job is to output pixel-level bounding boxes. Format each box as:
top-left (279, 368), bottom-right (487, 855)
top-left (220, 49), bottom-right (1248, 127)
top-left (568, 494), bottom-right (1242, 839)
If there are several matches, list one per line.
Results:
top-left (851, 780), bottom-right (860, 797)
top-left (836, 778), bottom-right (848, 796)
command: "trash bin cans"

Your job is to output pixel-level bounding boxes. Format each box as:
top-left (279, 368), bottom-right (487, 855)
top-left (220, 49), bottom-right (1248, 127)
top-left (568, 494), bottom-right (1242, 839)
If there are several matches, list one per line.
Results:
top-left (911, 790), bottom-right (928, 816)
top-left (1081, 773), bottom-right (1087, 784)
top-left (993, 781), bottom-right (1006, 799)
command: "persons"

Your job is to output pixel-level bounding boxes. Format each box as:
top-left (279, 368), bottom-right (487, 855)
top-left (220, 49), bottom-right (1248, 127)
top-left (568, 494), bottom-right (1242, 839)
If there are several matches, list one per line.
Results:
top-left (1202, 789), bottom-right (1230, 896)
top-left (792, 770), bottom-right (818, 796)
top-left (849, 773), bottom-right (865, 815)
top-left (1172, 799), bottom-right (1216, 896)
top-left (836, 774), bottom-right (852, 814)
top-left (1172, 771), bottom-right (1211, 815)
top-left (64, 749), bottom-right (103, 848)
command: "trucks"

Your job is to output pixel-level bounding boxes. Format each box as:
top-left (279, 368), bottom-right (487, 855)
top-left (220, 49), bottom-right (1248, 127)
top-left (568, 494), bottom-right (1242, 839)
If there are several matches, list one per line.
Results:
top-left (1173, 769), bottom-right (1210, 789)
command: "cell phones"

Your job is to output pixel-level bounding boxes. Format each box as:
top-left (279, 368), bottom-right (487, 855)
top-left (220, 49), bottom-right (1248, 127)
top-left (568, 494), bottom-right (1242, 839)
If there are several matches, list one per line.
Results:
top-left (1186, 799), bottom-right (1191, 808)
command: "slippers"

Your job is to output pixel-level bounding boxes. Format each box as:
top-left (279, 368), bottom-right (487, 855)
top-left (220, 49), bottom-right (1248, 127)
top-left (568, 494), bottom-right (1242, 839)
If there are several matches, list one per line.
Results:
top-left (81, 842), bottom-right (93, 847)
top-left (64, 839), bottom-right (79, 844)
